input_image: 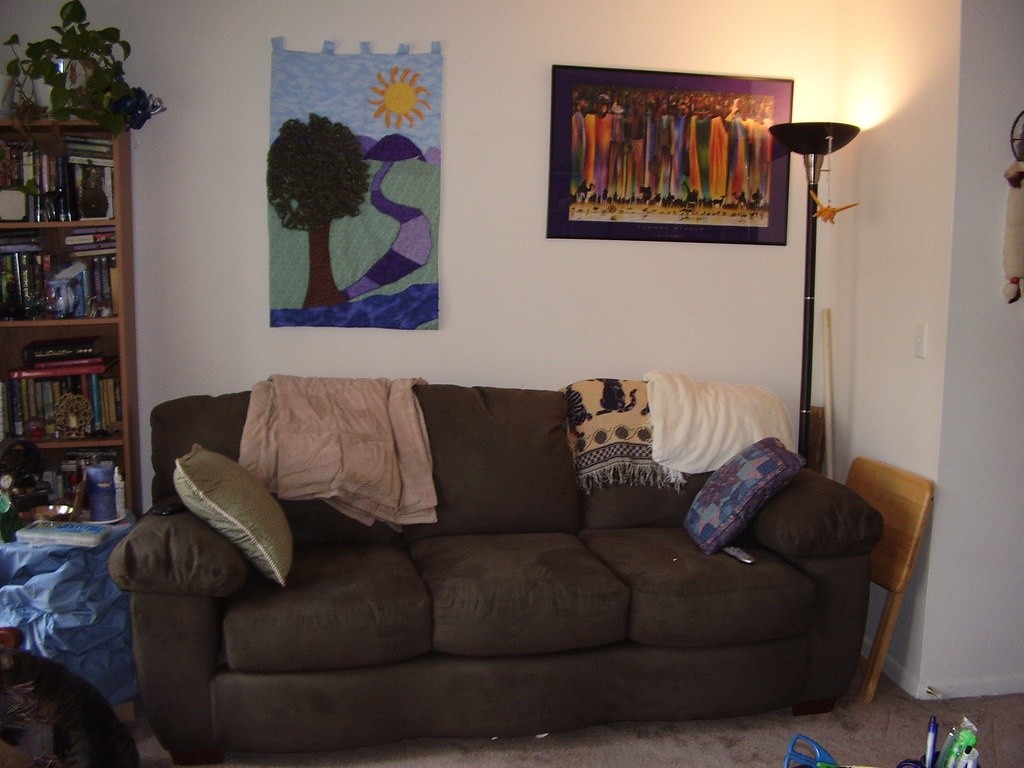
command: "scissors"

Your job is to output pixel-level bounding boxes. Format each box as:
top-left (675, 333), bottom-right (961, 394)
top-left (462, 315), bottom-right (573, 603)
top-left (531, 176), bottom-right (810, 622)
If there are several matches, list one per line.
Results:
top-left (784, 733), bottom-right (838, 768)
top-left (896, 758), bottom-right (923, 768)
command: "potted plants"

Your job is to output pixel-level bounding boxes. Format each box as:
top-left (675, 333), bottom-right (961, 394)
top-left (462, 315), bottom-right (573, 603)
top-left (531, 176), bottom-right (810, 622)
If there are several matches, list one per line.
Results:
top-left (3, 0), bottom-right (131, 139)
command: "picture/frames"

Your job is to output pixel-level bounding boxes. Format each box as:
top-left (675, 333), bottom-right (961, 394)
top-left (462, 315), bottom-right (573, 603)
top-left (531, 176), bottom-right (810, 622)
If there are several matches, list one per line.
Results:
top-left (546, 64), bottom-right (794, 245)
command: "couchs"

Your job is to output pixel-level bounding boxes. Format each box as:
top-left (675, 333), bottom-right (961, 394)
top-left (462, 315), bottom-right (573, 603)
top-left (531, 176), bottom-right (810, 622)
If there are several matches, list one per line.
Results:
top-left (109, 374), bottom-right (884, 768)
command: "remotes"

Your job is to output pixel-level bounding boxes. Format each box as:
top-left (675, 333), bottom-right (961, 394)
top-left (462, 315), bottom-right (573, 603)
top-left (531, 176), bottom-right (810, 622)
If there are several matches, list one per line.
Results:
top-left (723, 546), bottom-right (756, 564)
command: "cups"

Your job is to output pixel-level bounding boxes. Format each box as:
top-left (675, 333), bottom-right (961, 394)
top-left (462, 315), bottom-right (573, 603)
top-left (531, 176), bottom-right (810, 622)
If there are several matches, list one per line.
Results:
top-left (47, 278), bottom-right (79, 319)
top-left (88, 465), bottom-right (117, 521)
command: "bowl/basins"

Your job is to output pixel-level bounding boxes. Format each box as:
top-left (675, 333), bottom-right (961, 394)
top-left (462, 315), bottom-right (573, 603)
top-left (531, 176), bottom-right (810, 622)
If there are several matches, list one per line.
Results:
top-left (31, 505), bottom-right (74, 521)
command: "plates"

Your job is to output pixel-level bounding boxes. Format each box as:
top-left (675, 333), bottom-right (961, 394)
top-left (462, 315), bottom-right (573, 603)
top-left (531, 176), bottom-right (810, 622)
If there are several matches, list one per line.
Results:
top-left (80, 511), bottom-right (125, 526)
top-left (19, 435), bottom-right (51, 443)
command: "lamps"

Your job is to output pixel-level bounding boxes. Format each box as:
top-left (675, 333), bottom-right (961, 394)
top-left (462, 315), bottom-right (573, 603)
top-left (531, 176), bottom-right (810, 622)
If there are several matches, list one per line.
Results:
top-left (768, 122), bottom-right (860, 468)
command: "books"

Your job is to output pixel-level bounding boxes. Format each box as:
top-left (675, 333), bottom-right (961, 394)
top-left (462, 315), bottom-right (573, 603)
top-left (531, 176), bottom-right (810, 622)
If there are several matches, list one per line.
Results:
top-left (0, 136), bottom-right (124, 499)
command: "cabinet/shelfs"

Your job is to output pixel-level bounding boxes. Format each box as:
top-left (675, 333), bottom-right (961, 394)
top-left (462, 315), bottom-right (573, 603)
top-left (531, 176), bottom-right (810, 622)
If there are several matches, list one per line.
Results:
top-left (0, 118), bottom-right (142, 521)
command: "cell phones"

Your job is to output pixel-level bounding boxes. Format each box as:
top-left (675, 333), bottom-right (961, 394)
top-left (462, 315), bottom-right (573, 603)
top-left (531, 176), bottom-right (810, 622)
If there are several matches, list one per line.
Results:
top-left (153, 503), bottom-right (188, 515)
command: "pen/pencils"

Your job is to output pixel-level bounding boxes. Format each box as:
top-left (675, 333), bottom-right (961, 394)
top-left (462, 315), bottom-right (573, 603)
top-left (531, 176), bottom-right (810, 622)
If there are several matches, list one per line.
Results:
top-left (924, 716), bottom-right (979, 768)
top-left (816, 761), bottom-right (881, 768)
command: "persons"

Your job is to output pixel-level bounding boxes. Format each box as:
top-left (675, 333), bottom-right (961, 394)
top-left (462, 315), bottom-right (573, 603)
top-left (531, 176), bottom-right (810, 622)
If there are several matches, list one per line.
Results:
top-left (0, 645), bottom-right (139, 768)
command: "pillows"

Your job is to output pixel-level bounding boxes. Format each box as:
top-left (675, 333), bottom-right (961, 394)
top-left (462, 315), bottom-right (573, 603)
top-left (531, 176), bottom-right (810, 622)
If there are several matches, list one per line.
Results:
top-left (173, 442), bottom-right (294, 585)
top-left (683, 437), bottom-right (805, 556)
